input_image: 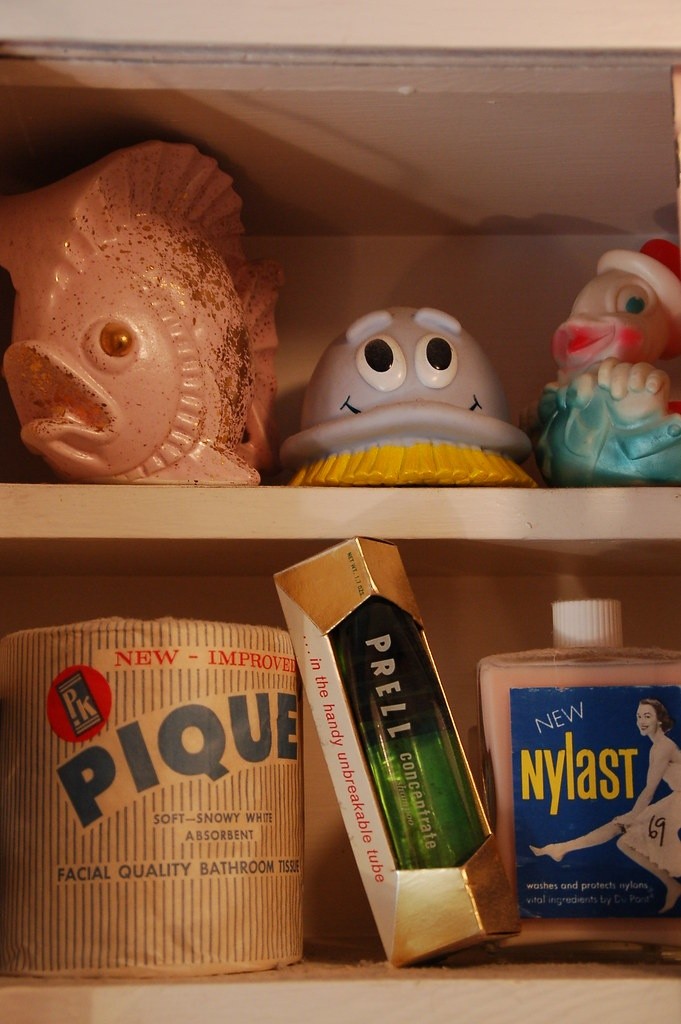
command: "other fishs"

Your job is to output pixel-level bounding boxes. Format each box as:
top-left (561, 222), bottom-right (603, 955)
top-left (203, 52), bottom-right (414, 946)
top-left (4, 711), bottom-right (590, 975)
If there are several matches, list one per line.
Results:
top-left (0, 138), bottom-right (280, 486)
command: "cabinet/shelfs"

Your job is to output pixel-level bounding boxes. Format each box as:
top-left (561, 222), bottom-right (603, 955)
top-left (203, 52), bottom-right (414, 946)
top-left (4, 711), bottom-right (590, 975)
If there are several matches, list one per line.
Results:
top-left (0, 0), bottom-right (681, 1024)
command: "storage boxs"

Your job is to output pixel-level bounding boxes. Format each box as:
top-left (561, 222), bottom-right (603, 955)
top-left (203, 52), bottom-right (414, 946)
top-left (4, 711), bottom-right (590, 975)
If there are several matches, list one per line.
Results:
top-left (273, 536), bottom-right (520, 966)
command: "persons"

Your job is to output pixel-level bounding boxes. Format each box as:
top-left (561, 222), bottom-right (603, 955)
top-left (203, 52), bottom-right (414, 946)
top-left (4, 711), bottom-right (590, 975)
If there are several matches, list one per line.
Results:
top-left (529, 699), bottom-right (681, 914)
